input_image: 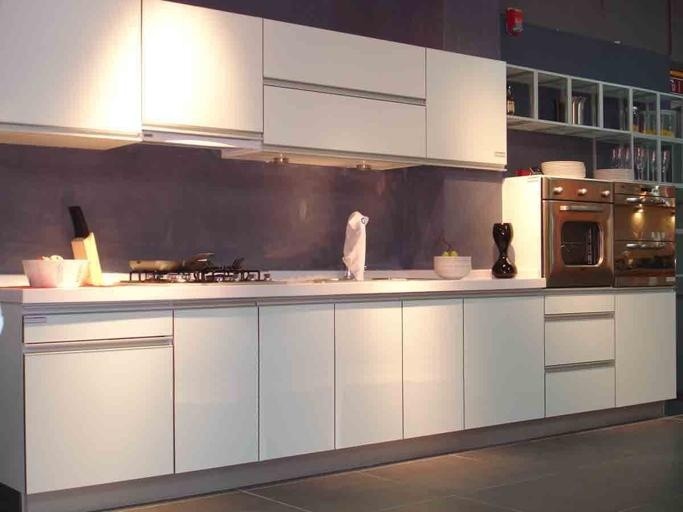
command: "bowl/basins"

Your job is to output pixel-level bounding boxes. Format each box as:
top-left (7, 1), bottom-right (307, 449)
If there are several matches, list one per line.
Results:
top-left (435, 256), bottom-right (473, 280)
top-left (21, 255), bottom-right (90, 289)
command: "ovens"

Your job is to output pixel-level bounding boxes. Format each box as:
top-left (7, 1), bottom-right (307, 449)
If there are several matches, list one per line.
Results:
top-left (539, 177), bottom-right (612, 288)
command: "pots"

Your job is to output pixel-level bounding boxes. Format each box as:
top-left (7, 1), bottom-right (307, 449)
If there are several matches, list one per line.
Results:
top-left (129, 252), bottom-right (216, 270)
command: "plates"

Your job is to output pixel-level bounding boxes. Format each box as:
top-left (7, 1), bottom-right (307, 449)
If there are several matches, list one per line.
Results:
top-left (541, 160), bottom-right (586, 178)
top-left (593, 169), bottom-right (634, 182)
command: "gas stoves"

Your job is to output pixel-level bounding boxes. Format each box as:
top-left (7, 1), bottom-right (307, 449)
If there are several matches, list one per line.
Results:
top-left (121, 268), bottom-right (285, 285)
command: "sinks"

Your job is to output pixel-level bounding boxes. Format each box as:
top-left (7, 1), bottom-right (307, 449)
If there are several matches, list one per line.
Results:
top-left (307, 277), bottom-right (445, 283)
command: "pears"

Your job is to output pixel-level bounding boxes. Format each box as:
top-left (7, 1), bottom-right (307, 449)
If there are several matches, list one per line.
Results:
top-left (442, 251), bottom-right (449, 256)
top-left (450, 250), bottom-right (458, 257)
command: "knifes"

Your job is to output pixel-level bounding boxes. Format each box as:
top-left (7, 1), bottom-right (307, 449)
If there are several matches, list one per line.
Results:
top-left (68, 206), bottom-right (92, 239)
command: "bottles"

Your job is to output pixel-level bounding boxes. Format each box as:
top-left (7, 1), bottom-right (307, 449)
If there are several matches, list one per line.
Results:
top-left (507, 86), bottom-right (515, 115)
top-left (625, 106), bottom-right (677, 137)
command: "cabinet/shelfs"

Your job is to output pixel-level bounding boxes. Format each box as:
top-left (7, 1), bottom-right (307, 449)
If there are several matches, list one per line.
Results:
top-left (174, 297), bottom-right (335, 499)
top-left (616, 292), bottom-right (677, 425)
top-left (426, 47), bottom-right (506, 173)
top-left (464, 296), bottom-right (546, 451)
top-left (334, 293), bottom-right (464, 473)
top-left (220, 18), bottom-right (426, 170)
top-left (506, 64), bottom-right (683, 190)
top-left (142, 0), bottom-right (263, 152)
top-left (544, 291), bottom-right (615, 436)
top-left (0, 0), bottom-right (143, 151)
top-left (2, 302), bottom-right (174, 510)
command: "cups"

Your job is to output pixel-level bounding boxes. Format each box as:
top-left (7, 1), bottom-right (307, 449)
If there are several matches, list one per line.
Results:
top-left (555, 96), bottom-right (588, 125)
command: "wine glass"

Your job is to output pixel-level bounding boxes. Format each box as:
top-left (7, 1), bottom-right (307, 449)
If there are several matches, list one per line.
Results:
top-left (613, 146), bottom-right (671, 183)
